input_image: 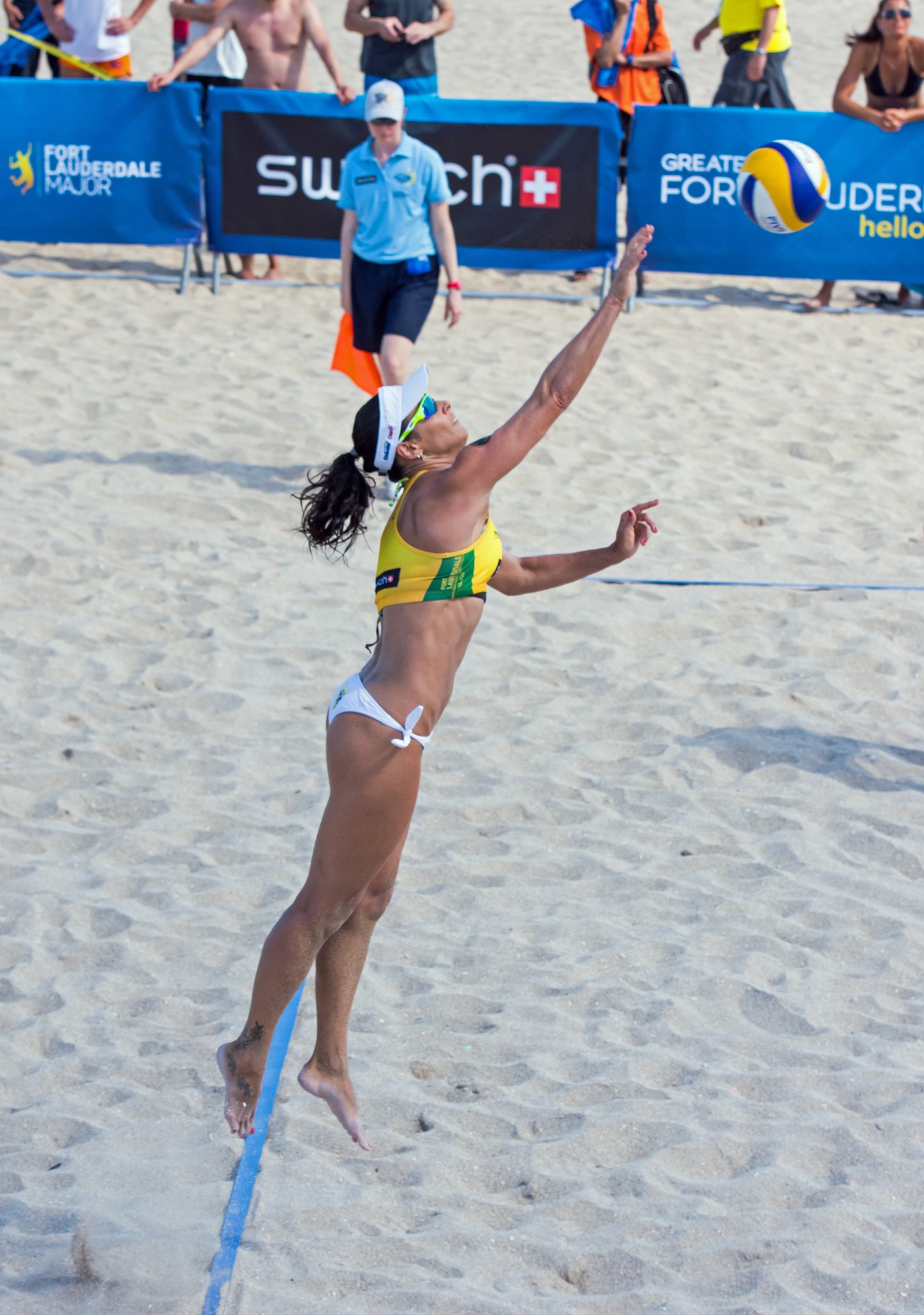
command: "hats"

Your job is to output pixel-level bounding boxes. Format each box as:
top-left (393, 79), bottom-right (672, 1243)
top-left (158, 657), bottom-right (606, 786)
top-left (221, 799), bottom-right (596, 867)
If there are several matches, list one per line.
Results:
top-left (373, 364), bottom-right (429, 476)
top-left (364, 78), bottom-right (405, 123)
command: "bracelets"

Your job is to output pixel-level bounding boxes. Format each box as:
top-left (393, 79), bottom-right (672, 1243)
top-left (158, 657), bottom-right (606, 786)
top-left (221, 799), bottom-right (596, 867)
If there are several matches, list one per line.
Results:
top-left (754, 49), bottom-right (767, 54)
top-left (625, 53), bottom-right (634, 65)
top-left (447, 281), bottom-right (460, 289)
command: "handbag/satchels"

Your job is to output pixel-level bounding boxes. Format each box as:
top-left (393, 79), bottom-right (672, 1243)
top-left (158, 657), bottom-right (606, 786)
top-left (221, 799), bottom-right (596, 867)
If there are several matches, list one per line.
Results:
top-left (720, 33), bottom-right (744, 56)
top-left (657, 65), bottom-right (689, 104)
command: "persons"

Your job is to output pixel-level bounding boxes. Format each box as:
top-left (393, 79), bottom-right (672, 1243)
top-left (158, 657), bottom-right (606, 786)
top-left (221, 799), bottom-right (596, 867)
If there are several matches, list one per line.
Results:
top-left (144, 0), bottom-right (357, 281)
top-left (343, 0), bottom-right (455, 95)
top-left (214, 223), bottom-right (660, 1152)
top-left (802, 0), bottom-right (924, 311)
top-left (570, 0), bottom-right (674, 281)
top-left (4, 0), bottom-right (157, 80)
top-left (692, 0), bottom-right (797, 111)
top-left (168, 0), bottom-right (248, 117)
top-left (334, 78), bottom-right (463, 386)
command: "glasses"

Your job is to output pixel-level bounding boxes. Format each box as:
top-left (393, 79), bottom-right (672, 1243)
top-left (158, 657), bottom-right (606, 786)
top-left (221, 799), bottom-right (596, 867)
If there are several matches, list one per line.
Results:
top-left (371, 118), bottom-right (396, 126)
top-left (880, 10), bottom-right (912, 20)
top-left (397, 393), bottom-right (438, 444)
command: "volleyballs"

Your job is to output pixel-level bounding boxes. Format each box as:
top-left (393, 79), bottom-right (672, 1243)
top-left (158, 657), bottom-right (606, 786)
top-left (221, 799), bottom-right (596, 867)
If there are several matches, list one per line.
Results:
top-left (735, 139), bottom-right (832, 235)
